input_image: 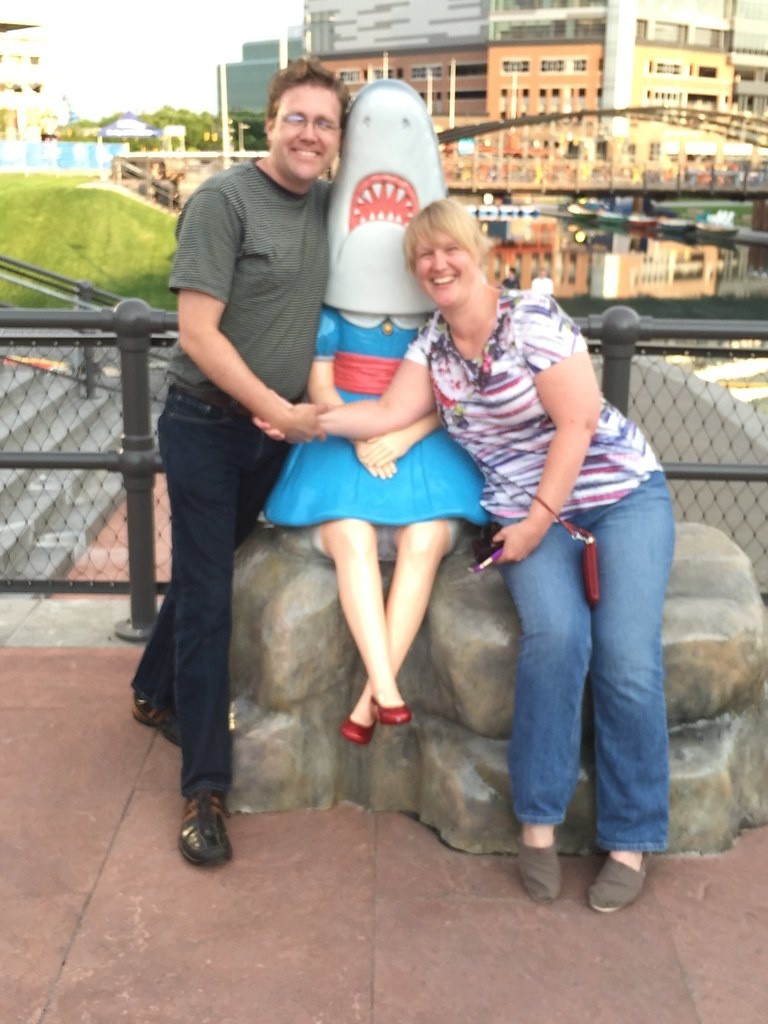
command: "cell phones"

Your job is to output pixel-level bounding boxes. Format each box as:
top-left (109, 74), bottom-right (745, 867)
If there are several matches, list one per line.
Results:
top-left (467, 543), bottom-right (504, 572)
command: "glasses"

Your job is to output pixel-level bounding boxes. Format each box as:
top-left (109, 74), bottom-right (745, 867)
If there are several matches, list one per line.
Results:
top-left (272, 112), bottom-right (342, 133)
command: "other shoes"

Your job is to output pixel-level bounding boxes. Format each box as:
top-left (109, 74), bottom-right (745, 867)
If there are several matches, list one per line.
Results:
top-left (341, 702), bottom-right (378, 745)
top-left (589, 851), bottom-right (651, 913)
top-left (518, 830), bottom-right (561, 901)
top-left (371, 696), bottom-right (411, 723)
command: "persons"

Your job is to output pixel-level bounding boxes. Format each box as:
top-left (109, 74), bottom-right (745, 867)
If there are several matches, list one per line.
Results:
top-left (132, 56), bottom-right (352, 871)
top-left (254, 195), bottom-right (680, 915)
top-left (260, 75), bottom-right (493, 748)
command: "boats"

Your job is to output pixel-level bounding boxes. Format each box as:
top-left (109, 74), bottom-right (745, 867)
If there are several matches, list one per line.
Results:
top-left (566, 202), bottom-right (739, 242)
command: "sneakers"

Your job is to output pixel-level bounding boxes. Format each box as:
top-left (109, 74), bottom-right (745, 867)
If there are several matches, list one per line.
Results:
top-left (133, 692), bottom-right (182, 745)
top-left (179, 787), bottom-right (232, 863)
